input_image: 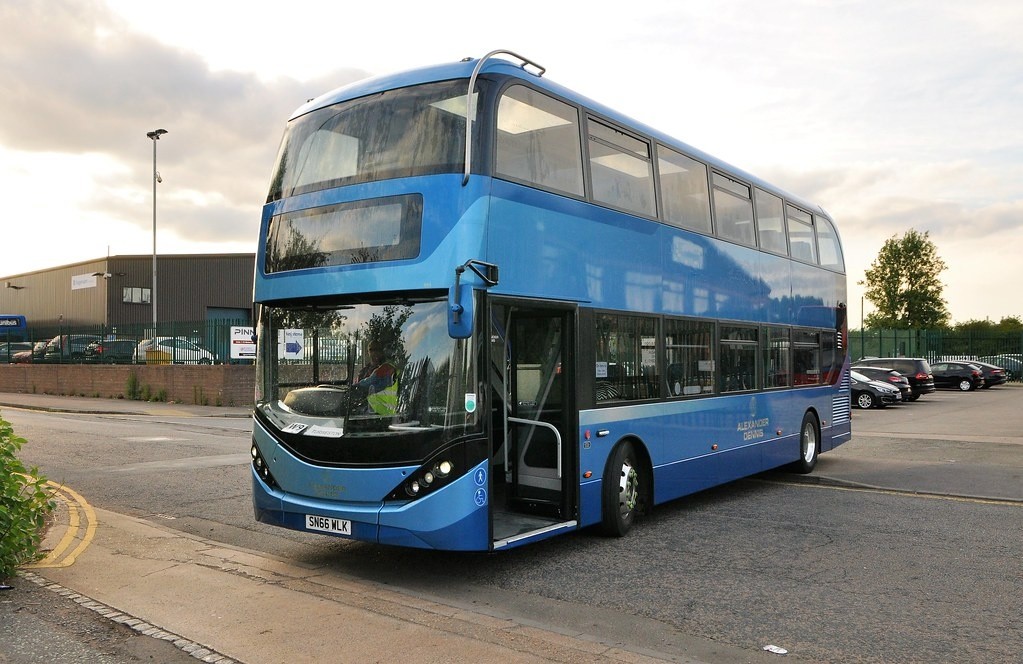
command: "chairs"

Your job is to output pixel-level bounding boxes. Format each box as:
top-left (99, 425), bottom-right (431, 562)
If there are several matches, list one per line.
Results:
top-left (512, 152), bottom-right (815, 264)
top-left (598, 349), bottom-right (815, 397)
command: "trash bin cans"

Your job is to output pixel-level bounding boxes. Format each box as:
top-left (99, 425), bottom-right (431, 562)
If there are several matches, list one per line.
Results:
top-left (145, 350), bottom-right (172, 365)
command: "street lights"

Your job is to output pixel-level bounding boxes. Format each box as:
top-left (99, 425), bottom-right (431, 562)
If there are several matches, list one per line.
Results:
top-left (146, 129), bottom-right (170, 336)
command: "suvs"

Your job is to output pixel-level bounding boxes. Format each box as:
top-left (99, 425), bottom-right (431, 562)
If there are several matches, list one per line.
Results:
top-left (854, 357), bottom-right (935, 401)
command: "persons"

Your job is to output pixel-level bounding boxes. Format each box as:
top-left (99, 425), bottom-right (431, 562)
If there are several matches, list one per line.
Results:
top-left (352, 339), bottom-right (401, 415)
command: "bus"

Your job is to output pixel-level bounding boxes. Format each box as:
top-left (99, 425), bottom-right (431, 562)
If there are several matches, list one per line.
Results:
top-left (250, 49), bottom-right (855, 553)
top-left (0, 315), bottom-right (29, 342)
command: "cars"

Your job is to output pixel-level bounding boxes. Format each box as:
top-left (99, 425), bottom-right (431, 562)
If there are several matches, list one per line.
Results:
top-left (849, 370), bottom-right (902, 409)
top-left (11, 352), bottom-right (33, 363)
top-left (929, 361), bottom-right (984, 391)
top-left (131, 337), bottom-right (215, 365)
top-left (962, 361), bottom-right (1006, 390)
top-left (45, 334), bottom-right (101, 364)
top-left (34, 339), bottom-right (54, 358)
top-left (87, 340), bottom-right (140, 365)
top-left (978, 356), bottom-right (1023, 382)
top-left (852, 368), bottom-right (911, 399)
top-left (0, 342), bottom-right (34, 362)
